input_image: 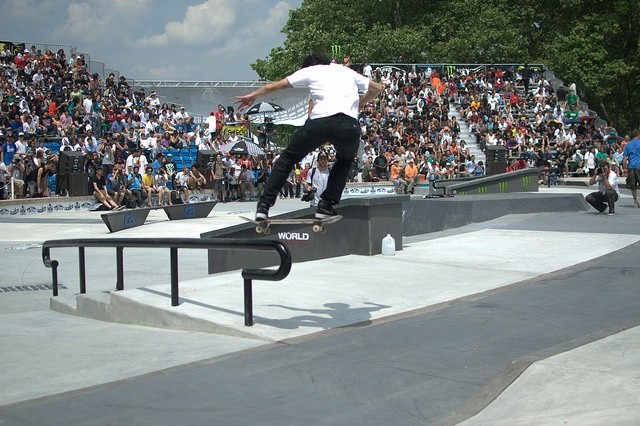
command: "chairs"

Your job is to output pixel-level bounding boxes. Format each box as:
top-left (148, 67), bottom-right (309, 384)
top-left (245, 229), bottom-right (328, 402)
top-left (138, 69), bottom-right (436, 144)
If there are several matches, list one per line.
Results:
top-left (42, 142), bottom-right (59, 154)
top-left (159, 141), bottom-right (198, 171)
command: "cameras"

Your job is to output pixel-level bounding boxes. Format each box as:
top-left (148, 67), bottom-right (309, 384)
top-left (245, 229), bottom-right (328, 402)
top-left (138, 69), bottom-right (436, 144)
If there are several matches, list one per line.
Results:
top-left (301, 187), bottom-right (317, 202)
top-left (159, 170), bottom-right (163, 174)
top-left (597, 168), bottom-right (604, 173)
top-left (25, 160), bottom-right (29, 164)
top-left (118, 168), bottom-right (122, 174)
top-left (14, 158), bottom-right (20, 164)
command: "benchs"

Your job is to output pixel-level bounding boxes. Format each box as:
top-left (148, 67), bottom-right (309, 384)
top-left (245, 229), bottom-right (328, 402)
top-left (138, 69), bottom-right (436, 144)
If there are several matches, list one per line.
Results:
top-left (511, 85), bottom-right (537, 123)
top-left (501, 141), bottom-right (579, 179)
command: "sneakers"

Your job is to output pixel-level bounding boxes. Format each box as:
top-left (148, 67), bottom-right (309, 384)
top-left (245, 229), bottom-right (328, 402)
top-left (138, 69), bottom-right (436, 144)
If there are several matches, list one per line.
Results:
top-left (315, 207), bottom-right (338, 219)
top-left (256, 209), bottom-right (268, 221)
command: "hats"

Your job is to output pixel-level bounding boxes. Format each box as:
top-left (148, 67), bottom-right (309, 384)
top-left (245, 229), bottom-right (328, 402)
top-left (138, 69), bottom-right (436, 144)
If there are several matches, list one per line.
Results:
top-left (318, 151), bottom-right (327, 157)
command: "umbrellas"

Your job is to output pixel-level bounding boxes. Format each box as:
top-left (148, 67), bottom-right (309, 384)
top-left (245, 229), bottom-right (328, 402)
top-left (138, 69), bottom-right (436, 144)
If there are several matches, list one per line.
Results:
top-left (244, 100), bottom-right (285, 118)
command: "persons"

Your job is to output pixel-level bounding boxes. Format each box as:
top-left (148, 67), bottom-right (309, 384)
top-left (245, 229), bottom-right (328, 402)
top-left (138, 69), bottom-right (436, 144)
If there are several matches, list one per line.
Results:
top-left (351, 61), bottom-right (444, 139)
top-left (154, 166), bottom-right (173, 206)
top-left (91, 72), bottom-right (194, 175)
top-left (189, 166), bottom-right (206, 192)
top-left (127, 166), bottom-right (148, 207)
top-left (207, 152), bottom-right (278, 201)
top-left (91, 167), bottom-right (122, 211)
top-left (195, 104), bottom-right (250, 152)
top-left (280, 147), bottom-right (312, 198)
top-left (324, 139), bottom-right (445, 195)
top-left (586, 108), bottom-right (628, 177)
top-left (233, 54), bottom-right (382, 222)
top-left (118, 168), bottom-right (132, 208)
top-left (586, 161), bottom-right (620, 216)
top-left (622, 130), bottom-right (640, 209)
top-left (304, 152), bottom-right (330, 207)
top-left (106, 166), bottom-right (126, 209)
top-left (256, 118), bottom-right (272, 148)
top-left (141, 167), bottom-right (162, 208)
top-left (0, 41), bottom-right (91, 200)
top-left (444, 61), bottom-right (586, 185)
top-left (312, 148), bottom-right (320, 168)
top-left (174, 167), bottom-right (190, 203)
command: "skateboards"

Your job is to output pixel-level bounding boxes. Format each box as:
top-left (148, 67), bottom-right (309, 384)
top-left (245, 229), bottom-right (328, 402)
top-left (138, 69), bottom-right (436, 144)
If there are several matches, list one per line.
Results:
top-left (239, 213), bottom-right (344, 235)
